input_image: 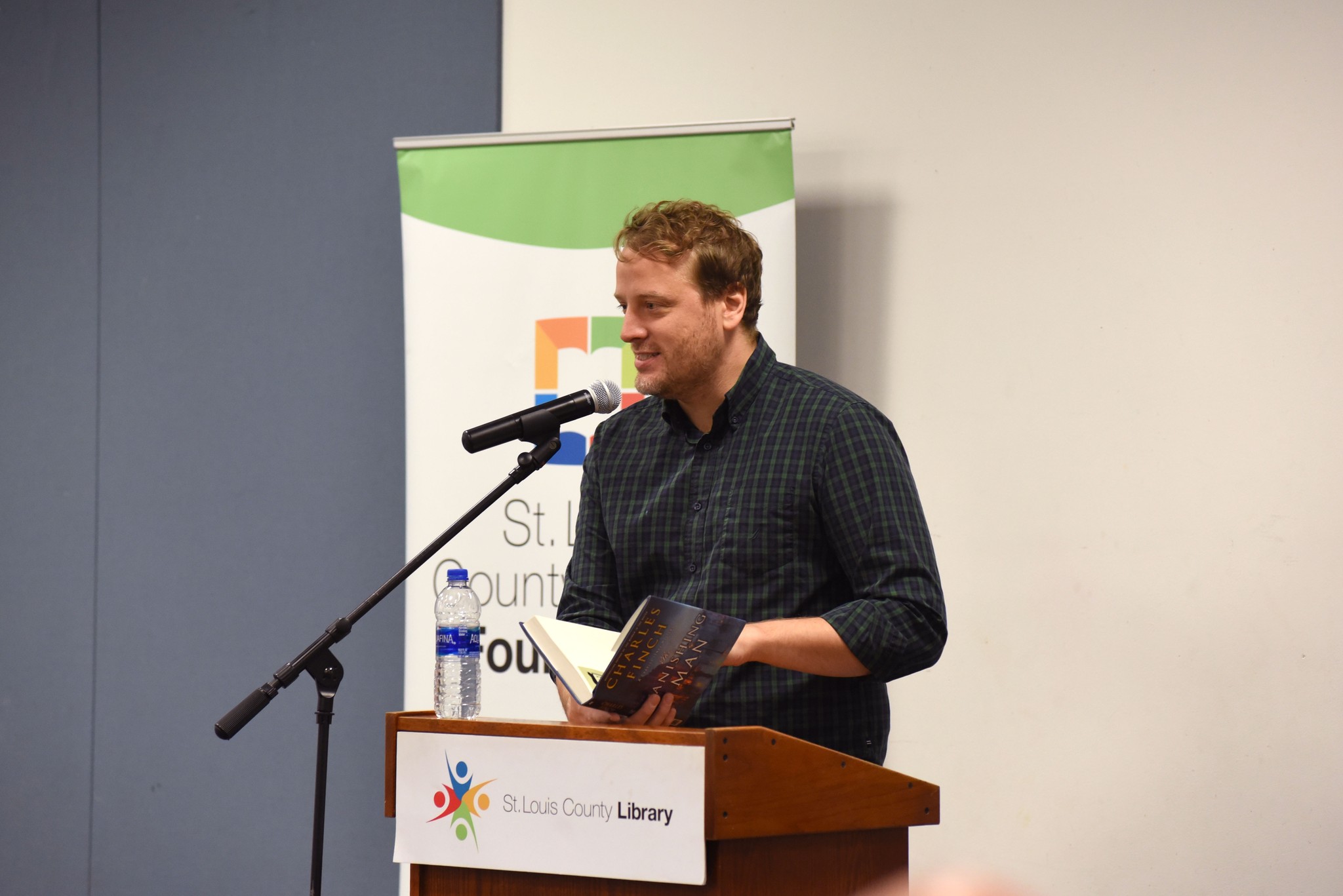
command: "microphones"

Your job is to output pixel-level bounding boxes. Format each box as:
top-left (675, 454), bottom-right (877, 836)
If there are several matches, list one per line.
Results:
top-left (462, 378), bottom-right (622, 454)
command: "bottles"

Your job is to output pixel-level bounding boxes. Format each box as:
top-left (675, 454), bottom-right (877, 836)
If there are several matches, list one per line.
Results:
top-left (434, 570), bottom-right (481, 720)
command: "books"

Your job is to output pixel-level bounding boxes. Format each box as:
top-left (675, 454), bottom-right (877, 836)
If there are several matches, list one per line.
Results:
top-left (518, 595), bottom-right (747, 727)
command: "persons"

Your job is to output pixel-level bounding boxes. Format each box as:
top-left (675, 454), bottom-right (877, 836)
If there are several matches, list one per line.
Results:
top-left (547, 197), bottom-right (949, 766)
